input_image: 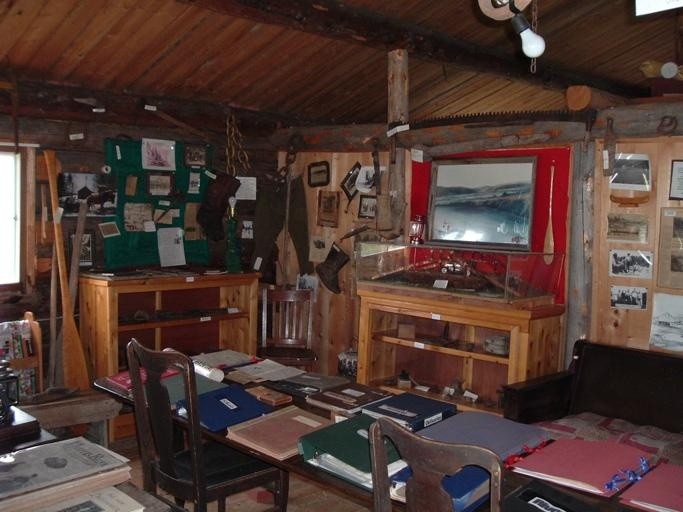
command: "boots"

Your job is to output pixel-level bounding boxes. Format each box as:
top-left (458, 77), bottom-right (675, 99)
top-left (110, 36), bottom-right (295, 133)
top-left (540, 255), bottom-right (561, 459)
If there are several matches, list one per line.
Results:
top-left (197, 170), bottom-right (241, 242)
top-left (315, 242), bottom-right (349, 293)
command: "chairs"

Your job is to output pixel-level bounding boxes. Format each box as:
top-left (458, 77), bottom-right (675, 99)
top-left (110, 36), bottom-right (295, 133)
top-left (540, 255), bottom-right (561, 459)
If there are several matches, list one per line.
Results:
top-left (258, 285), bottom-right (318, 372)
top-left (367, 417), bottom-right (503, 512)
top-left (126, 338), bottom-right (290, 510)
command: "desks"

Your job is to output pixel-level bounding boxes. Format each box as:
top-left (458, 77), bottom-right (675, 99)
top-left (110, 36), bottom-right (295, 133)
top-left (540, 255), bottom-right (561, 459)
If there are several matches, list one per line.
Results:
top-left (89, 347), bottom-right (653, 511)
top-left (0, 427), bottom-right (185, 512)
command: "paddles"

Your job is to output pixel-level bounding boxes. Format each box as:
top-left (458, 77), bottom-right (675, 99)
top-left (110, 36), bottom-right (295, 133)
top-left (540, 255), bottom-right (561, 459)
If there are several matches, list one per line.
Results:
top-left (43, 150), bottom-right (93, 393)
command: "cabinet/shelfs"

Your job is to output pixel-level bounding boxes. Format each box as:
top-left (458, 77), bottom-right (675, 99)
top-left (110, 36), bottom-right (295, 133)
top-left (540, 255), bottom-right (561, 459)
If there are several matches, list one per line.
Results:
top-left (78, 268), bottom-right (261, 382)
top-left (353, 277), bottom-right (566, 418)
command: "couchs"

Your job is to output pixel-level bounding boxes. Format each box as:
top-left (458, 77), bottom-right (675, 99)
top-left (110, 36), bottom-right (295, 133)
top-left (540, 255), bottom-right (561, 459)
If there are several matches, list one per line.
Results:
top-left (501, 338), bottom-right (682, 464)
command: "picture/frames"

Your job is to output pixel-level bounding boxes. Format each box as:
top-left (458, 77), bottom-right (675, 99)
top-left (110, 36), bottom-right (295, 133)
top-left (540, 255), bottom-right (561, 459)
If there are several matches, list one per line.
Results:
top-left (66, 228), bottom-right (97, 272)
top-left (655, 206), bottom-right (683, 296)
top-left (423, 152), bottom-right (539, 253)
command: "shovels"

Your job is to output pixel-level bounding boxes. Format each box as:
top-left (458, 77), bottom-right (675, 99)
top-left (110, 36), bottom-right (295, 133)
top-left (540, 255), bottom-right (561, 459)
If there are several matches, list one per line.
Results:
top-left (543, 166), bottom-right (554, 265)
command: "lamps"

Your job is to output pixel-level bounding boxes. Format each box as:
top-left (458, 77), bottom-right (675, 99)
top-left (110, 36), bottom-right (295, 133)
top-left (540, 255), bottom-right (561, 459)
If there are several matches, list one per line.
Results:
top-left (507, 0), bottom-right (546, 59)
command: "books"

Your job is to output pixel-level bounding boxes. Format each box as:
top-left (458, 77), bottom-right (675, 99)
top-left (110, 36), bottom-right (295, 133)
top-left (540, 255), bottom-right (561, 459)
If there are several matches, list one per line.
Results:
top-left (0, 319), bottom-right (36, 403)
